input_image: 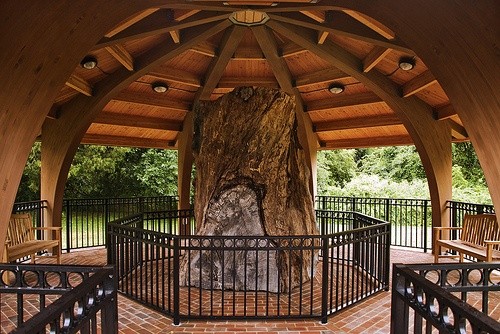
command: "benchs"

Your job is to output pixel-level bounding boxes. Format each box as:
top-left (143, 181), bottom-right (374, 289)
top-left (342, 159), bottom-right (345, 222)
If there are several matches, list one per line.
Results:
top-left (432, 213), bottom-right (500, 262)
top-left (2, 213), bottom-right (61, 285)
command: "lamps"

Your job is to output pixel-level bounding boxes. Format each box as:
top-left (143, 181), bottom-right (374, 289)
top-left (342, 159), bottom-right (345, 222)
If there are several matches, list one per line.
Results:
top-left (80, 54), bottom-right (97, 70)
top-left (397, 57), bottom-right (416, 72)
top-left (152, 82), bottom-right (168, 94)
top-left (329, 83), bottom-right (345, 95)
